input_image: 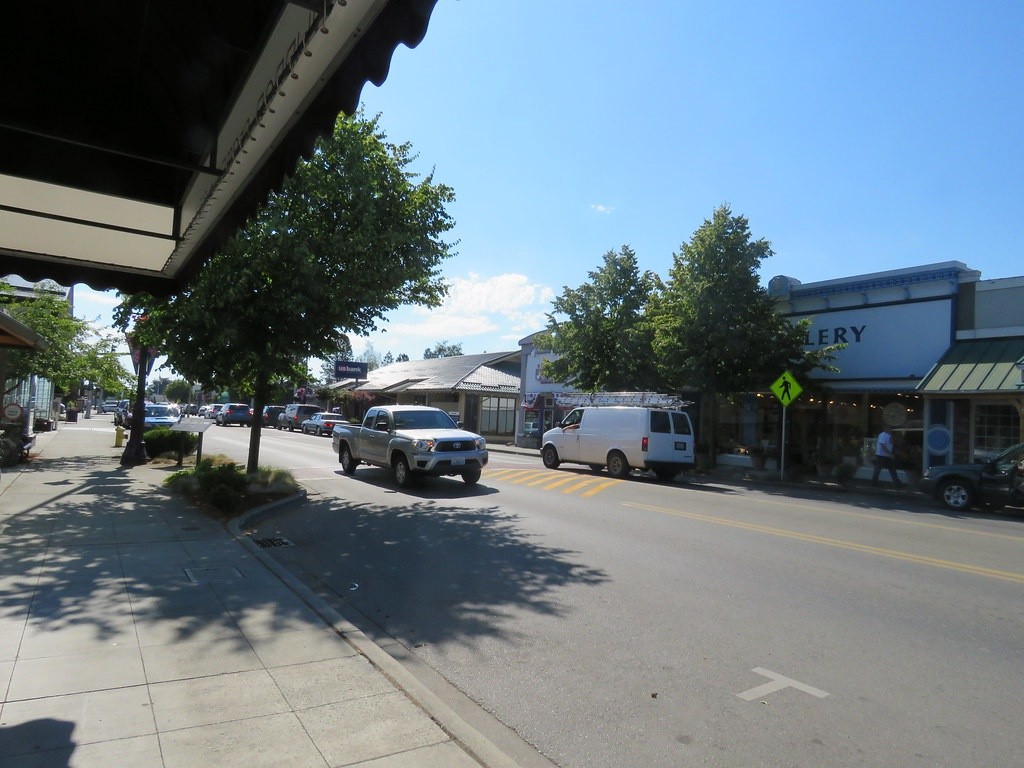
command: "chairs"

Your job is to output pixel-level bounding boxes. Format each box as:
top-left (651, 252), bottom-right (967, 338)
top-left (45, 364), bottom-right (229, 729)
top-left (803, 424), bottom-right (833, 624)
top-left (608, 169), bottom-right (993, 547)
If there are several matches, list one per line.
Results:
top-left (371, 431), bottom-right (388, 440)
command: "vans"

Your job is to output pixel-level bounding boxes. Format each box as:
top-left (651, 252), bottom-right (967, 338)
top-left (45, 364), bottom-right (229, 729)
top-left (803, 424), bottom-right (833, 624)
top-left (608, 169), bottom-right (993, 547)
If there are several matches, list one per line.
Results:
top-left (540, 406), bottom-right (694, 478)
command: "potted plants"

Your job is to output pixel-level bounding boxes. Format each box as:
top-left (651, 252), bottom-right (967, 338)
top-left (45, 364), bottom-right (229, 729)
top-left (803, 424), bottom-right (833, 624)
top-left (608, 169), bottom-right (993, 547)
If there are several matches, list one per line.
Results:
top-left (814, 454), bottom-right (838, 477)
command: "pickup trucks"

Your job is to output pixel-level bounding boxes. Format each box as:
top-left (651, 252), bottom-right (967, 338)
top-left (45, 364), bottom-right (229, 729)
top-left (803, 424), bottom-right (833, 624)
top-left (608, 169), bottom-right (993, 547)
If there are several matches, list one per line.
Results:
top-left (332, 405), bottom-right (488, 487)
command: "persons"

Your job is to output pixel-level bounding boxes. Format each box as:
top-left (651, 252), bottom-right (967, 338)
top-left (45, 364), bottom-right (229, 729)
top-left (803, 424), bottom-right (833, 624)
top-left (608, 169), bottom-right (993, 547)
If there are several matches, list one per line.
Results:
top-left (871, 426), bottom-right (905, 488)
top-left (562, 422), bottom-right (580, 434)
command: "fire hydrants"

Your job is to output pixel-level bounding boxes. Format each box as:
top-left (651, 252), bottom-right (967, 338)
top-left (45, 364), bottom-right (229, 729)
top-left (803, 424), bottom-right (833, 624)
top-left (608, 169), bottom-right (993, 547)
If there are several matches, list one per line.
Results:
top-left (115, 427), bottom-right (128, 447)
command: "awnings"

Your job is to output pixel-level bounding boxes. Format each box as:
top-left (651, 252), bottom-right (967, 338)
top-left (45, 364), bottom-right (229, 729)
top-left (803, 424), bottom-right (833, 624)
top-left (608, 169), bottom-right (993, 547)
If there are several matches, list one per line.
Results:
top-left (914, 336), bottom-right (1024, 399)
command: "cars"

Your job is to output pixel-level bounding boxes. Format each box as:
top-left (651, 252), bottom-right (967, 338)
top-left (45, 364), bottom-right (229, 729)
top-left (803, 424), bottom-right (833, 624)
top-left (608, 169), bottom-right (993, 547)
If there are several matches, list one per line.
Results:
top-left (102, 400), bottom-right (350, 437)
top-left (922, 443), bottom-right (1024, 511)
